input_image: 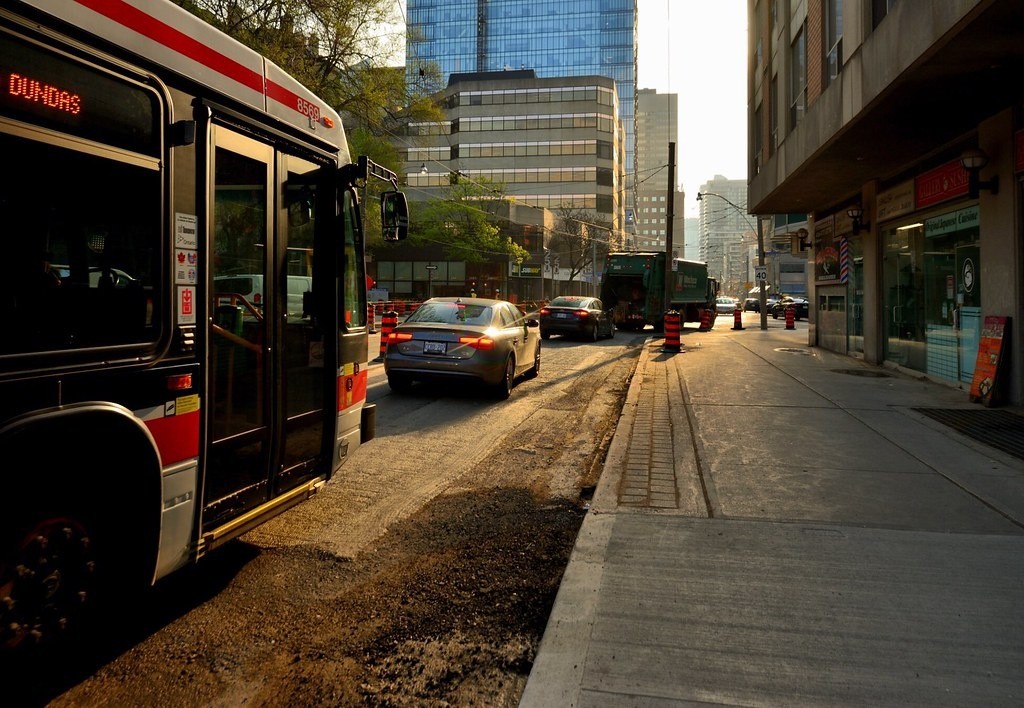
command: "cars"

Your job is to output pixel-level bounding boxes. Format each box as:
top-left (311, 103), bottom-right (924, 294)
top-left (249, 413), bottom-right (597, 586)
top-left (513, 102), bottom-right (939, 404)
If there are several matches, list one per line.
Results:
top-left (715, 298), bottom-right (737, 315)
top-left (766, 299), bottom-right (777, 315)
top-left (734, 299), bottom-right (742, 313)
top-left (539, 295), bottom-right (616, 342)
top-left (771, 295), bottom-right (810, 319)
top-left (742, 298), bottom-right (760, 312)
top-left (383, 297), bottom-right (542, 400)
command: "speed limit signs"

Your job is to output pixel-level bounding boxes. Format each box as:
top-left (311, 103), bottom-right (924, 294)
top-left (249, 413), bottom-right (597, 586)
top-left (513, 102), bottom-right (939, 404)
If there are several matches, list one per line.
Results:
top-left (756, 265), bottom-right (768, 281)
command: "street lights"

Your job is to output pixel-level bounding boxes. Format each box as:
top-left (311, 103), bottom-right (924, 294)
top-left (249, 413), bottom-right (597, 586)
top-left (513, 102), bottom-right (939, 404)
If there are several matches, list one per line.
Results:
top-left (695, 192), bottom-right (768, 330)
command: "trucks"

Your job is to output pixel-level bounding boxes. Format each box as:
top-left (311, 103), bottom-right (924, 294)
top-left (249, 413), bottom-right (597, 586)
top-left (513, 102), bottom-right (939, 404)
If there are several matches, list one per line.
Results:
top-left (600, 249), bottom-right (720, 332)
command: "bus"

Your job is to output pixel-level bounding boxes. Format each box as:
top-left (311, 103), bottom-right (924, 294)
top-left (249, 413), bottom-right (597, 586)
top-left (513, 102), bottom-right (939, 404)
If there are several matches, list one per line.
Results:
top-left (0, 0), bottom-right (408, 687)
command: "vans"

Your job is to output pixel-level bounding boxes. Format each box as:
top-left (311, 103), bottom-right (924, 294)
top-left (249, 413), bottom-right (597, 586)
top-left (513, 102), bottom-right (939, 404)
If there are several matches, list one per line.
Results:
top-left (213, 275), bottom-right (313, 323)
